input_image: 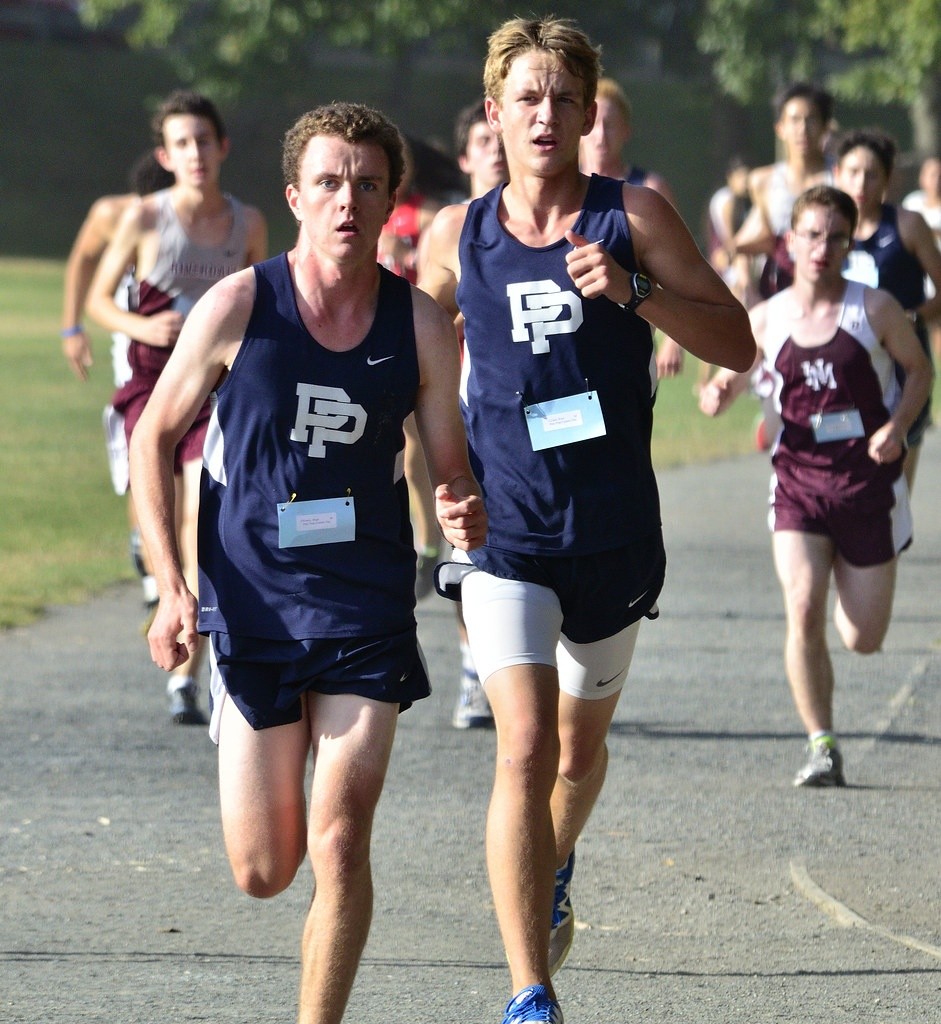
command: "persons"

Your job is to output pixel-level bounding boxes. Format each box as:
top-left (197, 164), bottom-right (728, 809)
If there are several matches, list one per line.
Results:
top-left (128, 101), bottom-right (485, 1024)
top-left (374, 96), bottom-right (509, 729)
top-left (684, 82), bottom-right (941, 787)
top-left (57, 97), bottom-right (269, 723)
top-left (408, 19), bottom-right (757, 1024)
top-left (578, 78), bottom-right (685, 411)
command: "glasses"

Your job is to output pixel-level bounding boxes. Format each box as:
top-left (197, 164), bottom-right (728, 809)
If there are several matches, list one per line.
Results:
top-left (795, 231), bottom-right (850, 248)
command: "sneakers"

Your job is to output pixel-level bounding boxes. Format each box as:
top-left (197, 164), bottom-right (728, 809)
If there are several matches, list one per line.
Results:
top-left (794, 743), bottom-right (843, 788)
top-left (501, 985), bottom-right (564, 1024)
top-left (168, 684), bottom-right (207, 725)
top-left (505, 847), bottom-right (575, 977)
top-left (453, 672), bottom-right (491, 728)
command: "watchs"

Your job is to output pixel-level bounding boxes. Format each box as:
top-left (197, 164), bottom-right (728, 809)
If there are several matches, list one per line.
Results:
top-left (617, 272), bottom-right (652, 314)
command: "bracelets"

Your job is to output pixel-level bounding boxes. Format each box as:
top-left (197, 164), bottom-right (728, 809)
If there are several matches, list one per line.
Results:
top-left (58, 324), bottom-right (83, 339)
